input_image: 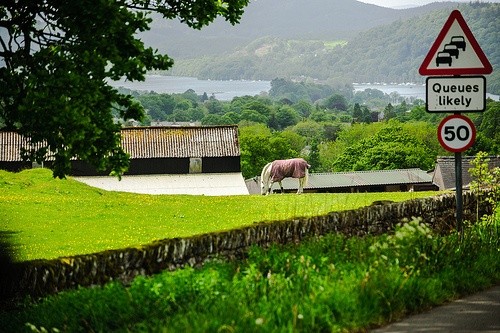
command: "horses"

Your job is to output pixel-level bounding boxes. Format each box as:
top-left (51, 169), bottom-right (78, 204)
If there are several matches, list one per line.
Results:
top-left (260, 158), bottom-right (310, 195)
